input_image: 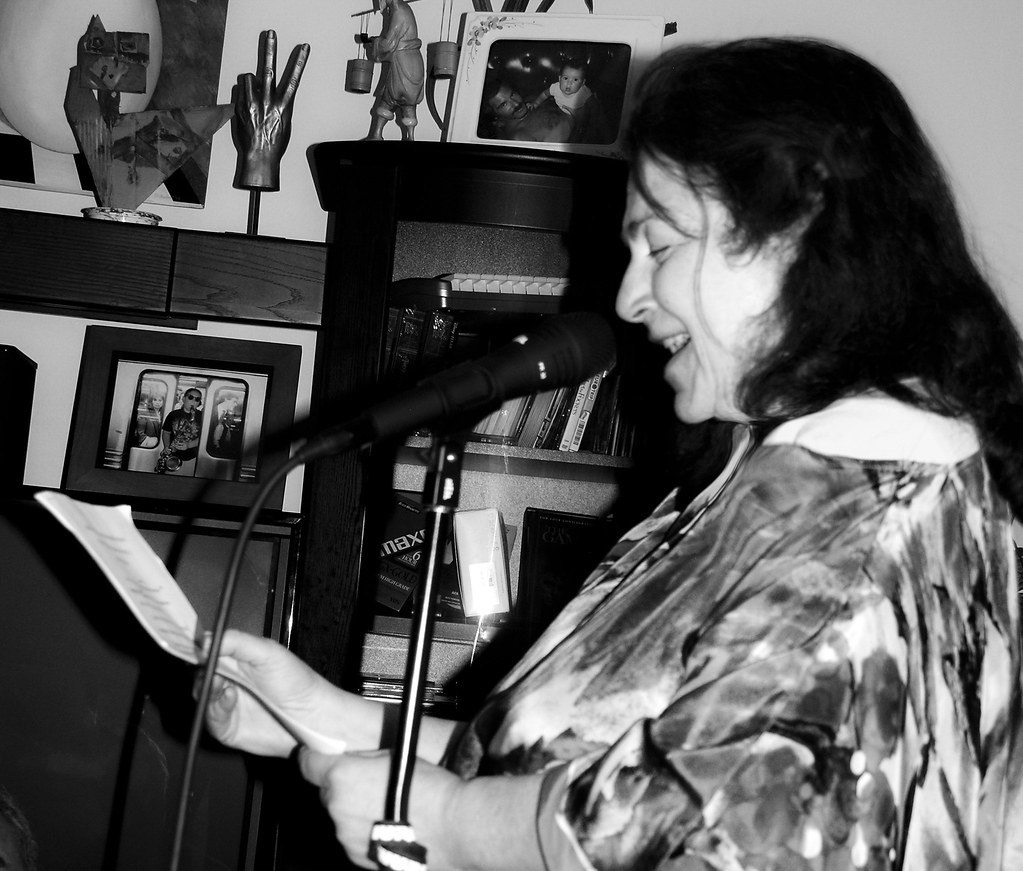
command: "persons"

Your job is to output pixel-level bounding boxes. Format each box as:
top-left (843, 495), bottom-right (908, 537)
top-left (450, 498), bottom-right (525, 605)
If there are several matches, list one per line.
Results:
top-left (195, 36), bottom-right (1023, 871)
top-left (231, 31), bottom-right (311, 192)
top-left (486, 55), bottom-right (618, 144)
top-left (355, 0), bottom-right (424, 140)
top-left (137, 386), bottom-right (239, 474)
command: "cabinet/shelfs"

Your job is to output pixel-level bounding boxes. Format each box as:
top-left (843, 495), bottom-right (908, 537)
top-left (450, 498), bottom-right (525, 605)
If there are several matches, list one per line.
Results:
top-left (263, 140), bottom-right (734, 871)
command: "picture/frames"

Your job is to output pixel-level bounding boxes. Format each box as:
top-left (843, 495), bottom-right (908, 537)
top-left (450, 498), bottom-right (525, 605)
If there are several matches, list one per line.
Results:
top-left (59, 325), bottom-right (303, 513)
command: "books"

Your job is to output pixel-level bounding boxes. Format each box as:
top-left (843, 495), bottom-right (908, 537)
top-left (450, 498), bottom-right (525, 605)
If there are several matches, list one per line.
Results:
top-left (477, 368), bottom-right (638, 458)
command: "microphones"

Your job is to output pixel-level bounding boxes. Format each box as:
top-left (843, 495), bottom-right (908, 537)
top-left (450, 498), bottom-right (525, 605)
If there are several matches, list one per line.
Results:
top-left (297, 311), bottom-right (618, 465)
